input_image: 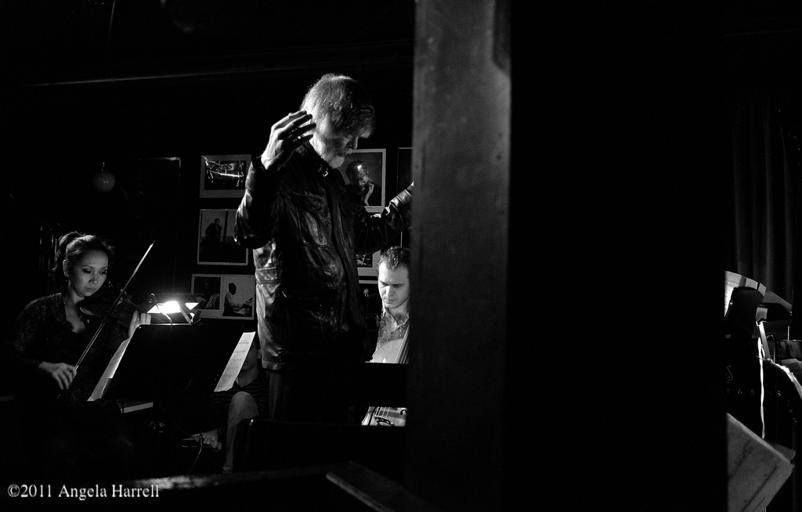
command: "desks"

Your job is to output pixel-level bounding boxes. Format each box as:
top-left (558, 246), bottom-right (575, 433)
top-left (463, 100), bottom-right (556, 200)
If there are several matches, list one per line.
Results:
top-left (0, 461), bottom-right (449, 512)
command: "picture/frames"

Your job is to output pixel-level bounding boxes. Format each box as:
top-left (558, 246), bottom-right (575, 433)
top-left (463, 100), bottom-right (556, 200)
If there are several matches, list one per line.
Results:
top-left (199, 154), bottom-right (251, 199)
top-left (356, 209), bottom-right (402, 284)
top-left (338, 148), bottom-right (387, 208)
top-left (197, 208), bottom-right (249, 266)
top-left (190, 274), bottom-right (256, 320)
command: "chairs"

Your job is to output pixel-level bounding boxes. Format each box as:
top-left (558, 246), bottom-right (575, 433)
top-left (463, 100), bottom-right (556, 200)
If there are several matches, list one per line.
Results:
top-left (725, 319), bottom-right (802, 512)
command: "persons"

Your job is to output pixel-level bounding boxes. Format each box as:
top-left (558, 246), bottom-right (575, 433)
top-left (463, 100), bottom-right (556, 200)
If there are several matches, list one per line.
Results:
top-left (14, 230), bottom-right (150, 479)
top-left (204, 218), bottom-right (222, 245)
top-left (231, 73), bottom-right (414, 428)
top-left (359, 246), bottom-right (413, 364)
top-left (342, 157), bottom-right (381, 206)
top-left (224, 281), bottom-right (246, 316)
top-left (198, 334), bottom-right (270, 469)
top-left (194, 278), bottom-right (219, 309)
